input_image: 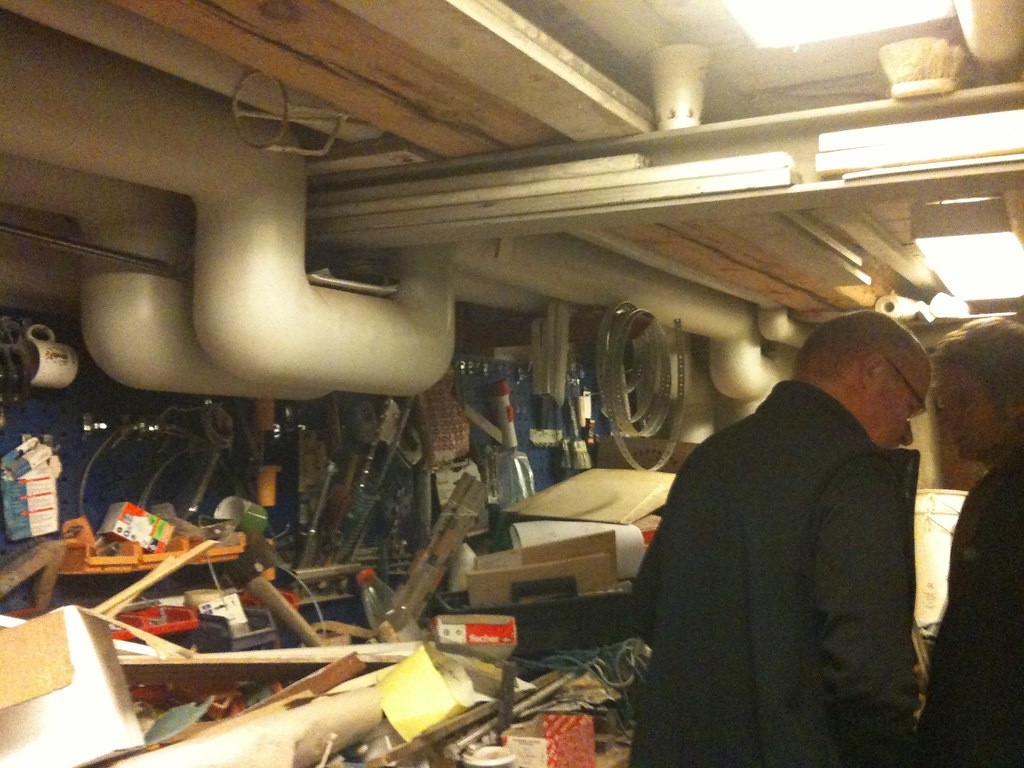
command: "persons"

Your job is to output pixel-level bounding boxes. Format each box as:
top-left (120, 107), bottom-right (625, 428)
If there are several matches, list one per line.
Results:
top-left (627, 310), bottom-right (932, 768)
top-left (914, 316), bottom-right (1024, 768)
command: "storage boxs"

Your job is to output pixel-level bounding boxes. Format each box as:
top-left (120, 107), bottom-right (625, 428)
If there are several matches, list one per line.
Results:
top-left (101, 591), bottom-right (355, 653)
top-left (0, 605), bottom-right (198, 768)
top-left (468, 528), bottom-right (619, 613)
top-left (500, 713), bottom-right (595, 767)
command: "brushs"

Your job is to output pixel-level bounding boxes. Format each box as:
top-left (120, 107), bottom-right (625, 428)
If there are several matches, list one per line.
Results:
top-left (559, 395), bottom-right (593, 471)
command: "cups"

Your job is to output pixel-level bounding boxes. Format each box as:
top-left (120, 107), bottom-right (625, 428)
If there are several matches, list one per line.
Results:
top-left (17, 323), bottom-right (80, 391)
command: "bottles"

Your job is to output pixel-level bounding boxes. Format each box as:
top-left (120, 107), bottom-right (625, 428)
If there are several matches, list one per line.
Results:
top-left (354, 566), bottom-right (421, 642)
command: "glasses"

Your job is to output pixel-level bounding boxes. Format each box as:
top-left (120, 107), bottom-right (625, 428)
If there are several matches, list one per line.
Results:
top-left (881, 355), bottom-right (927, 419)
